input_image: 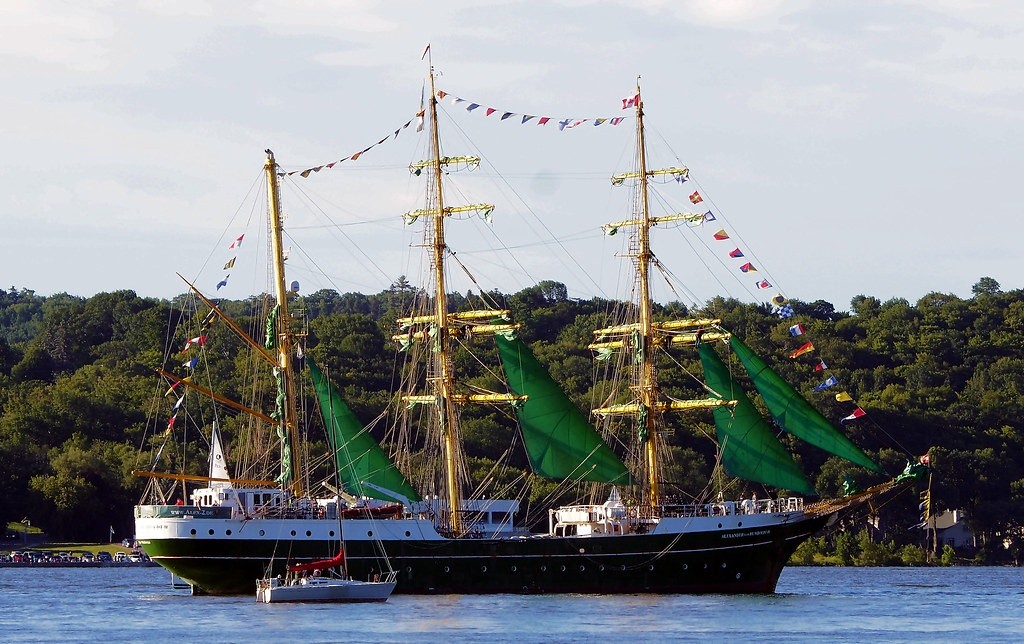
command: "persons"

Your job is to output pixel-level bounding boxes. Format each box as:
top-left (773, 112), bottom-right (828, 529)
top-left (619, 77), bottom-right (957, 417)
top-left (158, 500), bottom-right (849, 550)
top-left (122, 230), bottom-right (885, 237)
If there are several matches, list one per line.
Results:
top-left (293, 561), bottom-right (307, 586)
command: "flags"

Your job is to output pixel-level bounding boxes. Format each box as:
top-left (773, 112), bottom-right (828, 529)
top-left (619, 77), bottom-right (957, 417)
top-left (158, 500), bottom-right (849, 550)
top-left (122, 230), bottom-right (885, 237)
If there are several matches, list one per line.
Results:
top-left (277, 89), bottom-right (640, 181)
top-left (674, 169), bottom-right (867, 423)
top-left (166, 234), bottom-right (245, 438)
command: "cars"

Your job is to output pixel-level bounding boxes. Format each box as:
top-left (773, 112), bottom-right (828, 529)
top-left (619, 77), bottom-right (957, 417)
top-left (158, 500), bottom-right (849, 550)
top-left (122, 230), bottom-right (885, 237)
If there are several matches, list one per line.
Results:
top-left (0, 550), bottom-right (153, 564)
top-left (122, 538), bottom-right (134, 548)
top-left (133, 540), bottom-right (142, 549)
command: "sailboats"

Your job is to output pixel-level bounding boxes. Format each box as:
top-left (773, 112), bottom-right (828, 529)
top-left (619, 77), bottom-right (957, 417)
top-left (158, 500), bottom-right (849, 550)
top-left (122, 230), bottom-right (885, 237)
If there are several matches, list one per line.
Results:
top-left (127, 41), bottom-right (932, 598)
top-left (253, 360), bottom-right (400, 603)
